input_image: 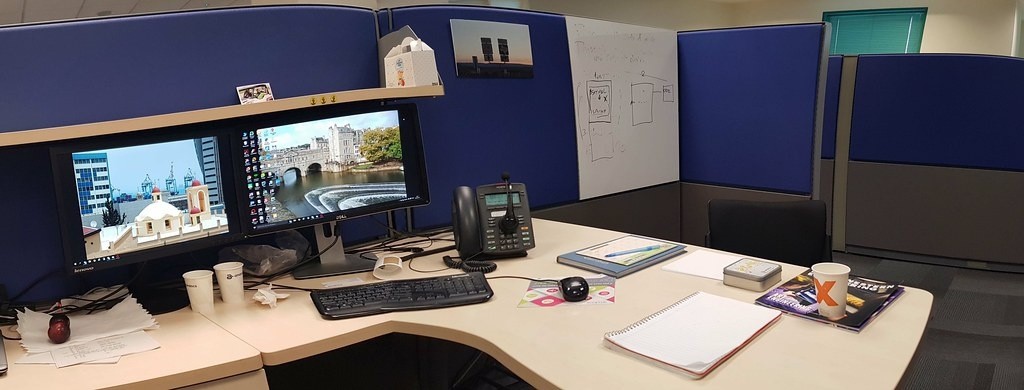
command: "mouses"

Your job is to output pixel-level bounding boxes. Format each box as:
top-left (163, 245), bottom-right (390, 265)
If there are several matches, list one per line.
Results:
top-left (559, 276), bottom-right (589, 302)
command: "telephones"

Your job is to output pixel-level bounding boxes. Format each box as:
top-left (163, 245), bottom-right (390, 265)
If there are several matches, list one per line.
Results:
top-left (449, 172), bottom-right (536, 262)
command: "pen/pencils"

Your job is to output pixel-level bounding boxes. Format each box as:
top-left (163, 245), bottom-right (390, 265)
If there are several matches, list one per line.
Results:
top-left (603, 244), bottom-right (660, 258)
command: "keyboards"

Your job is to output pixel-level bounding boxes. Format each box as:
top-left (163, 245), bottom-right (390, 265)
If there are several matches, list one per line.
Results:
top-left (310, 272), bottom-right (494, 320)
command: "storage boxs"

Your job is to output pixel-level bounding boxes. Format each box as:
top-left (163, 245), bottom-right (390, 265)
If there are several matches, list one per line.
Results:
top-left (385, 37), bottom-right (439, 88)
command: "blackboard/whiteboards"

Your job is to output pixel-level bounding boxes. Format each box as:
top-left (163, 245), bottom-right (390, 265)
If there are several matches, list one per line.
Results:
top-left (564, 12), bottom-right (680, 202)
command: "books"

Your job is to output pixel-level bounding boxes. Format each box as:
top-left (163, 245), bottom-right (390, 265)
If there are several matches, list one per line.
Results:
top-left (606, 291), bottom-right (783, 380)
top-left (757, 264), bottom-right (905, 331)
top-left (554, 231), bottom-right (690, 275)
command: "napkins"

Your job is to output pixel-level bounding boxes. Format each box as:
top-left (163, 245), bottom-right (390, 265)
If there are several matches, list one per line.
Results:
top-left (15, 294), bottom-right (160, 353)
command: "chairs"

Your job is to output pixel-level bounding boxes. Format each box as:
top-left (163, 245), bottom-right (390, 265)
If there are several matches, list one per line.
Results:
top-left (705, 199), bottom-right (832, 267)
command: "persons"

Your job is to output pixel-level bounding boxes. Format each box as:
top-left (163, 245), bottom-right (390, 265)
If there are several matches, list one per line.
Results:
top-left (243, 87), bottom-right (268, 99)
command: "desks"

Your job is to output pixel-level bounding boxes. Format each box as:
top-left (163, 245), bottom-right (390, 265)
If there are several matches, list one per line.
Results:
top-left (0, 307), bottom-right (269, 390)
top-left (195, 218), bottom-right (934, 390)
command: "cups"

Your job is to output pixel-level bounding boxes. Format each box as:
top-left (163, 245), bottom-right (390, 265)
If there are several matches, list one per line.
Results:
top-left (182, 270), bottom-right (214, 313)
top-left (373, 256), bottom-right (403, 280)
top-left (213, 262), bottom-right (244, 304)
top-left (811, 262), bottom-right (851, 318)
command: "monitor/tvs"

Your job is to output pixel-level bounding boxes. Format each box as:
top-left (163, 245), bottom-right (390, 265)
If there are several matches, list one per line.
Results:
top-left (230, 102), bottom-right (431, 280)
top-left (48, 127), bottom-right (248, 315)
